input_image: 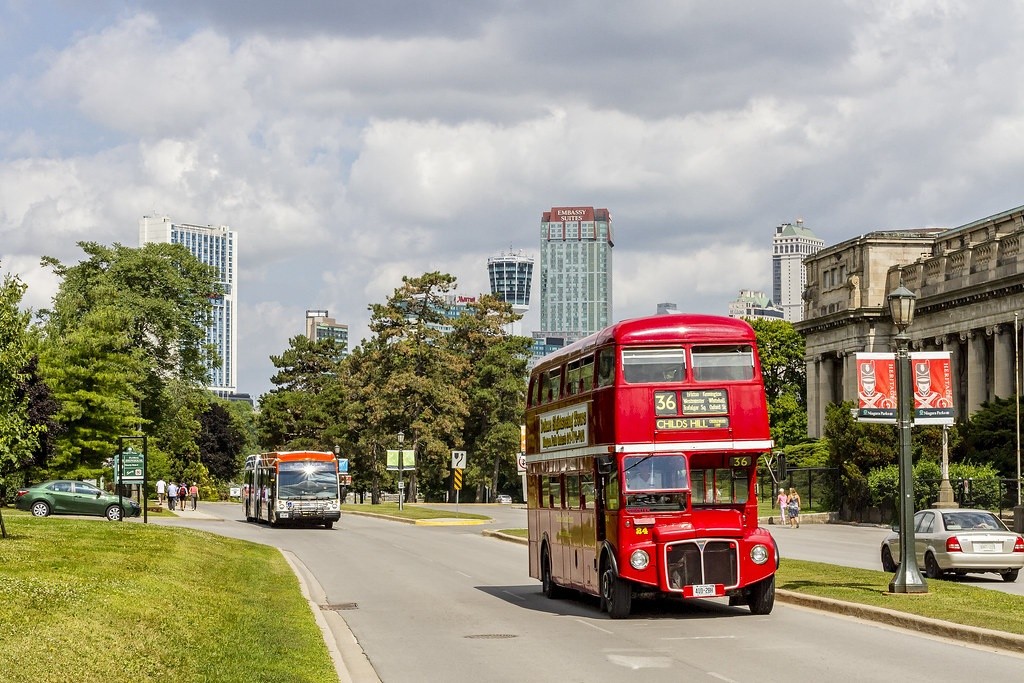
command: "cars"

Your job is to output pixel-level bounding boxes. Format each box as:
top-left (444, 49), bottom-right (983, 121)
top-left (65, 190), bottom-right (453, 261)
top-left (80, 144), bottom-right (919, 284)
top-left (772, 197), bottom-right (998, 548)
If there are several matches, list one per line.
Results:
top-left (16, 479), bottom-right (143, 522)
top-left (495, 494), bottom-right (513, 504)
top-left (879, 506), bottom-right (1024, 583)
top-left (381, 491), bottom-right (388, 500)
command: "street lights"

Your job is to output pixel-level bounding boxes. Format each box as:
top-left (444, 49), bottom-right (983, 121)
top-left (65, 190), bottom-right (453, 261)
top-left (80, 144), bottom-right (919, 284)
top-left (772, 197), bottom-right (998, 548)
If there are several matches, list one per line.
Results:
top-left (887, 277), bottom-right (929, 595)
top-left (335, 444), bottom-right (340, 460)
top-left (397, 430), bottom-right (405, 510)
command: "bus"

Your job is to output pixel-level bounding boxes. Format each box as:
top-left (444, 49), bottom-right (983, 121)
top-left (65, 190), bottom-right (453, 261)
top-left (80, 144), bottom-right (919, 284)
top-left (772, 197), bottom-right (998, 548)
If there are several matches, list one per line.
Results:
top-left (527, 313), bottom-right (787, 619)
top-left (242, 450), bottom-right (344, 529)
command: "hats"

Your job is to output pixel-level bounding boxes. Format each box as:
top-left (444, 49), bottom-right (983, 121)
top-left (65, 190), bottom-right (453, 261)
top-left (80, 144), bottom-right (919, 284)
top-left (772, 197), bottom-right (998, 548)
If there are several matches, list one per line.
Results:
top-left (779, 488), bottom-right (784, 492)
top-left (193, 482), bottom-right (196, 485)
top-left (180, 483), bottom-right (187, 486)
top-left (790, 488), bottom-right (796, 495)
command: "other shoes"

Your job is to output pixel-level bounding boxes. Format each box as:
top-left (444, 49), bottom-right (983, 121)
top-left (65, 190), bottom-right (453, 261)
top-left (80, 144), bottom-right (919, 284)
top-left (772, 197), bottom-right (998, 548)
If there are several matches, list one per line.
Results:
top-left (796, 524), bottom-right (799, 528)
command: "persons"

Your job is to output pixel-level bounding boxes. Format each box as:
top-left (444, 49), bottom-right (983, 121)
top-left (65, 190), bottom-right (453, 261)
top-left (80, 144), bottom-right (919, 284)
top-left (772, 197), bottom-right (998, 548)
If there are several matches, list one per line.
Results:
top-left (155, 477), bottom-right (199, 511)
top-left (707, 485), bottom-right (721, 502)
top-left (787, 488), bottom-right (801, 528)
top-left (629, 463), bottom-right (661, 490)
top-left (775, 488), bottom-right (787, 526)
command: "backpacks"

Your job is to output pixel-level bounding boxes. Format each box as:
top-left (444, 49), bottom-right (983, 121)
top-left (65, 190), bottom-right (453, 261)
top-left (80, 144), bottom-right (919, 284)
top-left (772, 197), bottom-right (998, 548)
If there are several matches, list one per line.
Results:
top-left (181, 487), bottom-right (185, 494)
top-left (192, 487), bottom-right (197, 494)
